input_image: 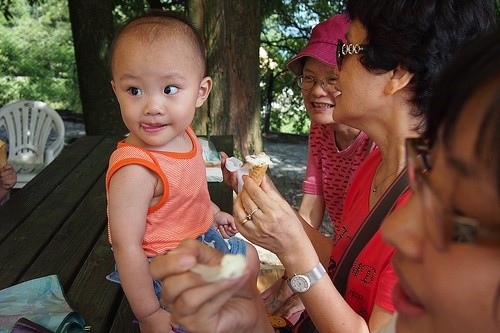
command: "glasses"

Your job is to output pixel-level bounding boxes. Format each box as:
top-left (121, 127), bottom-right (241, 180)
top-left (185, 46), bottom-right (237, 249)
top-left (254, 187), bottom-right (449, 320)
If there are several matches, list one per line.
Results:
top-left (335, 38), bottom-right (378, 73)
top-left (405, 136), bottom-right (500, 253)
top-left (297, 75), bottom-right (339, 93)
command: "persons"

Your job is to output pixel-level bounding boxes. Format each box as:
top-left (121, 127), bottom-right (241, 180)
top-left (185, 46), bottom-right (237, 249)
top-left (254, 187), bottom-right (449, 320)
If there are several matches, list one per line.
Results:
top-left (0, 141), bottom-right (17, 201)
top-left (219, 14), bottom-right (372, 240)
top-left (105, 15), bottom-right (260, 333)
top-left (148, 0), bottom-right (456, 333)
top-left (381, 0), bottom-right (500, 333)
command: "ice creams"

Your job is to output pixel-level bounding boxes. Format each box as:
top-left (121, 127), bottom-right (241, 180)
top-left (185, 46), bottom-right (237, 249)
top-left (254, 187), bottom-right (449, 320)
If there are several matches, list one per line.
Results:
top-left (219, 251), bottom-right (247, 280)
top-left (245, 152), bottom-right (271, 187)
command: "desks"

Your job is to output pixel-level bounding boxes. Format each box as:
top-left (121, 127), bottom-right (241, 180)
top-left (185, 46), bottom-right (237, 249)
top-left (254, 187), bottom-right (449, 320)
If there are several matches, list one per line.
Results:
top-left (0, 132), bottom-right (237, 333)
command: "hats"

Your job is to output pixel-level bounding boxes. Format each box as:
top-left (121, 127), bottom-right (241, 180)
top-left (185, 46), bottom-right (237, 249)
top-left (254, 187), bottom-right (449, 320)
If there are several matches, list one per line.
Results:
top-left (286, 14), bottom-right (352, 76)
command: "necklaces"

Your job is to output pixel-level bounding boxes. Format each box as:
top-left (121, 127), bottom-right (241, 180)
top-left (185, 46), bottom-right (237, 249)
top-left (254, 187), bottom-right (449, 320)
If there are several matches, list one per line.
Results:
top-left (372, 161), bottom-right (406, 192)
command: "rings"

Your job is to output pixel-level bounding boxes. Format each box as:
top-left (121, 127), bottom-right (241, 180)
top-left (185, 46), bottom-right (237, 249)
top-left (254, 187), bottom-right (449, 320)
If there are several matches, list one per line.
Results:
top-left (241, 219), bottom-right (248, 225)
top-left (246, 208), bottom-right (259, 220)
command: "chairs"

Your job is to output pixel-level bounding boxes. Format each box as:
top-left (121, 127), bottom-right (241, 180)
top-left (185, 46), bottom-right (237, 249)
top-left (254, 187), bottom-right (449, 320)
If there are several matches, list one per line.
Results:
top-left (0, 100), bottom-right (66, 189)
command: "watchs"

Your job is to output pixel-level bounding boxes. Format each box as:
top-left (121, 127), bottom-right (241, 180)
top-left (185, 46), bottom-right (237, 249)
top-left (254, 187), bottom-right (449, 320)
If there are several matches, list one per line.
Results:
top-left (286, 263), bottom-right (326, 293)
